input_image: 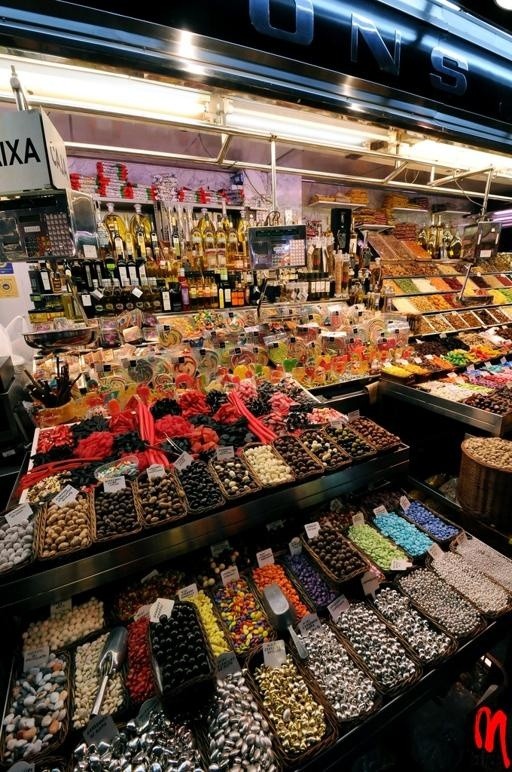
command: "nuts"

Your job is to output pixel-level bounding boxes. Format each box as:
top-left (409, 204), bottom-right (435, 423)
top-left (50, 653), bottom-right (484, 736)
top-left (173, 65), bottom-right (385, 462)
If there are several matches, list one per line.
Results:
top-left (379, 259), bottom-right (512, 334)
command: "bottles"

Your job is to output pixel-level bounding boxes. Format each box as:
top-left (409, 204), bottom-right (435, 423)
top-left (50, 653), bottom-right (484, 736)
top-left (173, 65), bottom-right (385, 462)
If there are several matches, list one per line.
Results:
top-left (297, 271), bottom-right (331, 302)
top-left (62, 253), bottom-right (148, 293)
top-left (334, 250), bottom-right (393, 313)
top-left (190, 207), bottom-right (251, 274)
top-left (101, 202), bottom-right (128, 250)
top-left (114, 225), bottom-right (182, 260)
top-left (429, 214), bottom-right (445, 248)
top-left (187, 269), bottom-right (260, 311)
top-left (39, 260), bottom-right (53, 294)
top-left (79, 267), bottom-right (190, 319)
top-left (128, 203), bottom-right (153, 247)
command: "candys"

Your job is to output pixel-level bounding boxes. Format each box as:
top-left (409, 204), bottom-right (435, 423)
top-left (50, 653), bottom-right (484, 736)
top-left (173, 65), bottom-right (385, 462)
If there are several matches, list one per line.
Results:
top-left (0, 304), bottom-right (512, 772)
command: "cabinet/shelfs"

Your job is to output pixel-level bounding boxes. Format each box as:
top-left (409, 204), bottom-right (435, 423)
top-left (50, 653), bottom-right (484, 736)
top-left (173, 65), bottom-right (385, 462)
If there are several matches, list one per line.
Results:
top-left (308, 201), bottom-right (471, 230)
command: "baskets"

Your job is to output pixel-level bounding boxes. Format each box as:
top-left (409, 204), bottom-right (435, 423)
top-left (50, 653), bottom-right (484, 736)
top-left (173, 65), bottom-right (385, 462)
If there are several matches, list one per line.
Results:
top-left (379, 342), bottom-right (512, 415)
top-left (0, 415), bottom-right (512, 772)
